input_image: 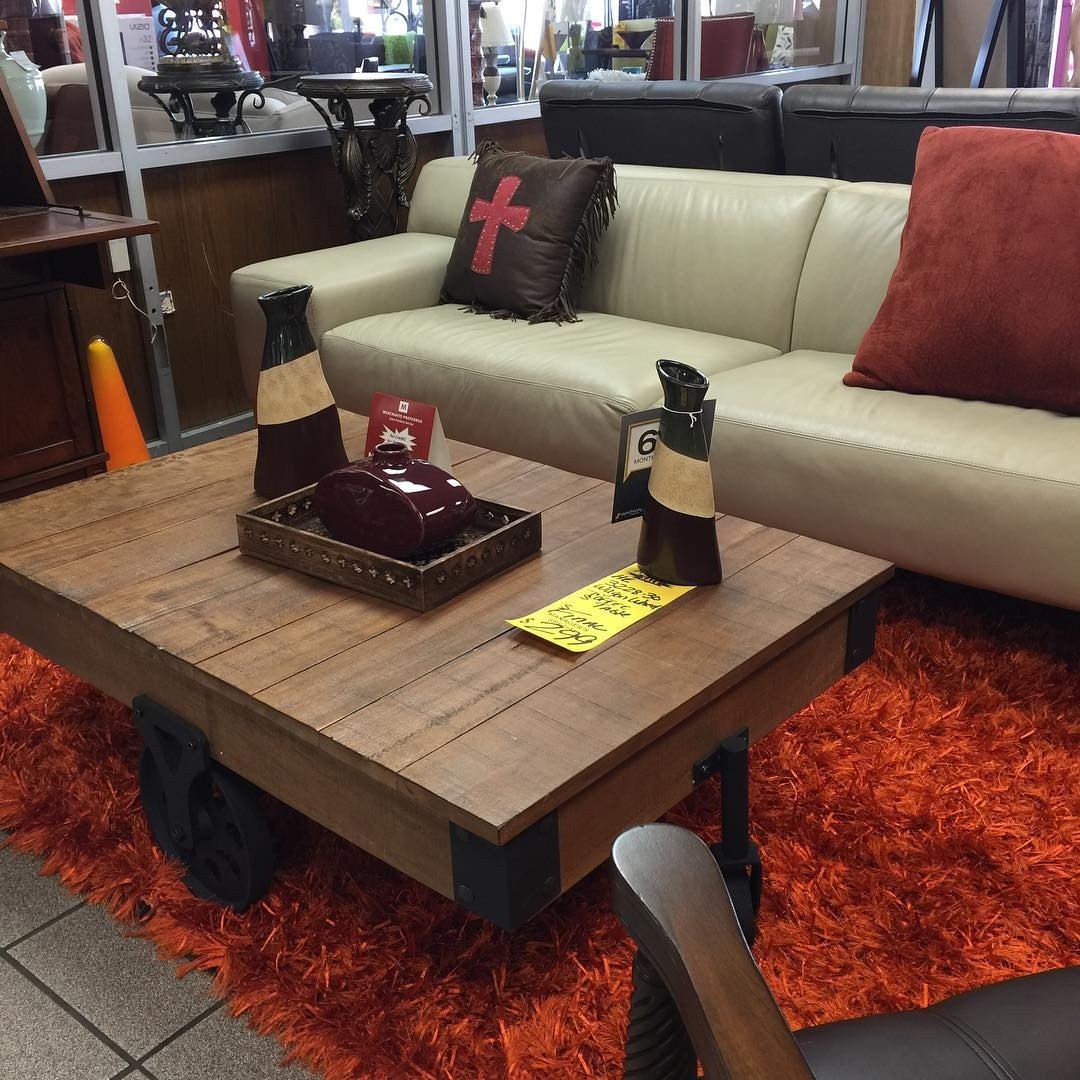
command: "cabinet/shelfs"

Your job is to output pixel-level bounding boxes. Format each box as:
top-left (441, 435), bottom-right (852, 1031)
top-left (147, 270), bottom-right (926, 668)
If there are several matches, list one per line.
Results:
top-left (0, 66), bottom-right (160, 501)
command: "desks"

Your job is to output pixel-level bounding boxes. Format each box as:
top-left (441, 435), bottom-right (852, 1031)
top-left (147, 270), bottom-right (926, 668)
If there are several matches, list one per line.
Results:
top-left (580, 49), bottom-right (652, 71)
top-left (296, 72), bottom-right (434, 238)
top-left (140, 71), bottom-right (265, 140)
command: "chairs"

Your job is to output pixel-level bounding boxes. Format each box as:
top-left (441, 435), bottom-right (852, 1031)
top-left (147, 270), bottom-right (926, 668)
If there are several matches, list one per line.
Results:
top-left (645, 12), bottom-right (756, 80)
top-left (609, 824), bottom-right (1080, 1080)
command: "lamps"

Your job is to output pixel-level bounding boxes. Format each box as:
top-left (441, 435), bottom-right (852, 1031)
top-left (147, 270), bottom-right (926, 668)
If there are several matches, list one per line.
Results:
top-left (479, 7), bottom-right (516, 106)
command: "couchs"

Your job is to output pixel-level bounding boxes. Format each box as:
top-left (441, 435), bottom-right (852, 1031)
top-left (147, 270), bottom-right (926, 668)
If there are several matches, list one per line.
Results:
top-left (43, 24), bottom-right (1080, 615)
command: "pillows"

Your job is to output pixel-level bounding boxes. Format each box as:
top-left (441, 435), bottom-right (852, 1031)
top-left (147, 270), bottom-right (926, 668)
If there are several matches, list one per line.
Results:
top-left (440, 138), bottom-right (620, 325)
top-left (382, 30), bottom-right (418, 65)
top-left (843, 125), bottom-right (1080, 419)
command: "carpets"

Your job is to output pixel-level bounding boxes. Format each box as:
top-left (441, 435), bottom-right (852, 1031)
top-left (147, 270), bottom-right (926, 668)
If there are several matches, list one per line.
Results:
top-left (0, 599), bottom-right (1080, 1080)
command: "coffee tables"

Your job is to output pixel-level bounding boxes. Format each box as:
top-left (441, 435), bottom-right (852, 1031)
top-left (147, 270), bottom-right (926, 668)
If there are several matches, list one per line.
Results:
top-left (0, 409), bottom-right (894, 947)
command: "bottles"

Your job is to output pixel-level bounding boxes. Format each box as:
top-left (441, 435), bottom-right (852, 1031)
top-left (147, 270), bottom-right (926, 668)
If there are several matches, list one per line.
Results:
top-left (254, 284), bottom-right (348, 499)
top-left (315, 441), bottom-right (479, 557)
top-left (638, 359), bottom-right (723, 586)
